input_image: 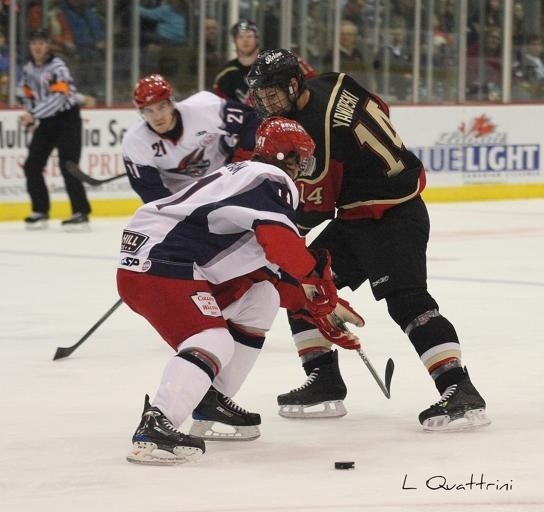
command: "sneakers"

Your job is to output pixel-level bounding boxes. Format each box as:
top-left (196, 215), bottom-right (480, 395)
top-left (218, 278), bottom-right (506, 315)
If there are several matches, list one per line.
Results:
top-left (23, 211), bottom-right (48, 224)
top-left (62, 213), bottom-right (88, 225)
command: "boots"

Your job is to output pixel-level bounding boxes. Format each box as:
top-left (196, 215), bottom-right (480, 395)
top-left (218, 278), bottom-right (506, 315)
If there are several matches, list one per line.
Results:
top-left (275, 349), bottom-right (347, 407)
top-left (417, 365), bottom-right (486, 425)
top-left (130, 393), bottom-right (205, 454)
top-left (191, 383), bottom-right (261, 426)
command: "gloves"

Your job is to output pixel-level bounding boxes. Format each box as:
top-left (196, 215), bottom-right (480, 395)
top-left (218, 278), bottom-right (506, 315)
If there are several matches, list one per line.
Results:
top-left (302, 249), bottom-right (337, 309)
top-left (305, 300), bottom-right (366, 350)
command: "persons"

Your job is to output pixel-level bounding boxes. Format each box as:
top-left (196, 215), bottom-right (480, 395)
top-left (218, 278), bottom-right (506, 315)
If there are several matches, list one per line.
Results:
top-left (119, 73), bottom-right (251, 204)
top-left (247, 48), bottom-right (486, 425)
top-left (113, 115), bottom-right (369, 451)
top-left (13, 29), bottom-right (91, 225)
top-left (0, 0), bottom-right (543, 109)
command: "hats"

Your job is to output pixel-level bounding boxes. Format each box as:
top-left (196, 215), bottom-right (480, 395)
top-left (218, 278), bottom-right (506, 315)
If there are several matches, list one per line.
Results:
top-left (28, 28), bottom-right (49, 41)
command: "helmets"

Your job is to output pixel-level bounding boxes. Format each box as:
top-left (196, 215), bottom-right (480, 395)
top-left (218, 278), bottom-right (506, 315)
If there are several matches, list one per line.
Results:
top-left (245, 48), bottom-right (303, 92)
top-left (230, 20), bottom-right (257, 36)
top-left (132, 73), bottom-right (172, 109)
top-left (254, 115), bottom-right (315, 162)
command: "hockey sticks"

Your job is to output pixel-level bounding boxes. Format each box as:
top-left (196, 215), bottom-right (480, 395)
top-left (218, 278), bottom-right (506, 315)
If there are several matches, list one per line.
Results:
top-left (313, 290), bottom-right (394, 399)
top-left (66, 161), bottom-right (127, 186)
top-left (47, 298), bottom-right (122, 361)
